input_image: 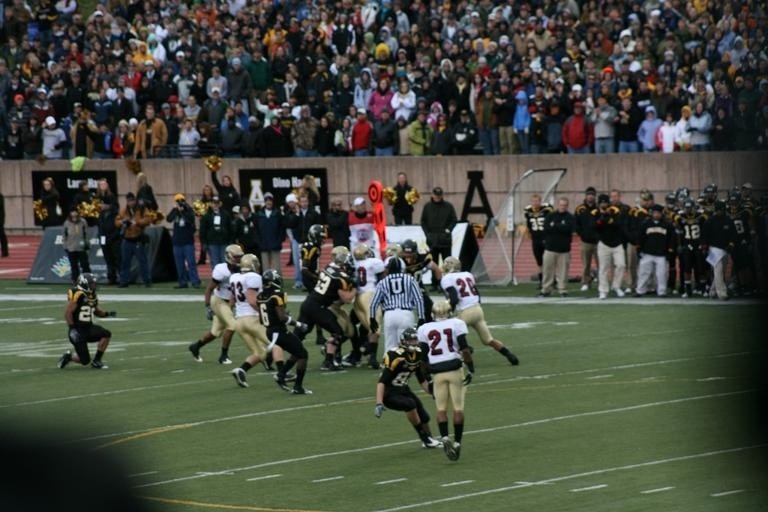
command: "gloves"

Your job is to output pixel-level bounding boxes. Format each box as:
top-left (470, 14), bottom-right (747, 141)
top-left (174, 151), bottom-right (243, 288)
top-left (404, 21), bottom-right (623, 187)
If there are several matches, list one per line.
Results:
top-left (205, 304), bottom-right (214, 322)
top-left (106, 310), bottom-right (116, 316)
top-left (370, 317), bottom-right (378, 334)
top-left (375, 402), bottom-right (385, 418)
top-left (299, 322), bottom-right (307, 331)
top-left (69, 326), bottom-right (79, 343)
top-left (417, 319), bottom-right (425, 329)
top-left (463, 373), bottom-right (473, 386)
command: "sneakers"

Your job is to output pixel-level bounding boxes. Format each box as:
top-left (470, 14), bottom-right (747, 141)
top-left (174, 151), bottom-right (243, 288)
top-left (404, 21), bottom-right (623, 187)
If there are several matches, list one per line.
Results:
top-left (368, 361), bottom-right (385, 370)
top-left (422, 442), bottom-right (444, 448)
top-left (57, 350), bottom-right (71, 369)
top-left (219, 356), bottom-right (233, 364)
top-left (91, 359), bottom-right (109, 369)
top-left (421, 437), bottom-right (439, 448)
top-left (442, 437), bottom-right (457, 461)
top-left (452, 441), bottom-right (461, 462)
top-left (614, 288), bottom-right (625, 297)
top-left (292, 386), bottom-right (313, 395)
top-left (189, 344), bottom-right (203, 362)
top-left (232, 367), bottom-right (249, 387)
top-left (319, 361), bottom-right (343, 371)
top-left (273, 375), bottom-right (289, 392)
top-left (343, 361), bottom-right (362, 367)
top-left (599, 291), bottom-right (607, 300)
top-left (282, 373), bottom-right (296, 381)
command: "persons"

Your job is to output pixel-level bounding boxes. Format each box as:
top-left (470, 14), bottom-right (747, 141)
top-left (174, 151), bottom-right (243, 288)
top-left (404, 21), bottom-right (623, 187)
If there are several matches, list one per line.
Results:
top-left (286, 197), bottom-right (321, 291)
top-left (114, 192), bottom-right (154, 289)
top-left (61, 208), bottom-right (91, 286)
top-left (36, 178), bottom-right (63, 227)
top-left (420, 187), bottom-right (457, 292)
top-left (699, 184), bottom-right (725, 216)
top-left (97, 198), bottom-right (117, 285)
top-left (649, 195), bottom-right (662, 212)
top-left (200, 196), bottom-right (234, 268)
top-left (256, 269), bottom-right (312, 395)
top-left (96, 179), bottom-right (112, 201)
top-left (195, 185), bottom-right (217, 266)
top-left (391, 172), bottom-right (416, 225)
top-left (73, 181), bottom-right (92, 210)
top-left (573, 187), bottom-right (601, 293)
top-left (662, 193), bottom-right (679, 217)
top-left (727, 191), bottom-right (751, 296)
top-left (344, 197), bottom-right (375, 259)
top-left (525, 195), bottom-right (555, 290)
top-left (383, 240), bottom-right (442, 331)
top-left (165, 193), bottom-right (202, 289)
top-left (375, 328), bottom-right (444, 450)
top-left (417, 299), bottom-right (476, 462)
top-left (210, 167), bottom-right (241, 213)
top-left (189, 245), bottom-right (244, 366)
top-left (274, 246), bottom-right (358, 393)
top-left (624, 191), bottom-right (653, 293)
top-left (740, 182), bottom-right (757, 210)
top-left (228, 254), bottom-right (296, 388)
top-left (633, 204), bottom-right (677, 298)
top-left (441, 256), bottom-right (520, 373)
top-left (300, 224), bottom-right (328, 344)
top-left (136, 173), bottom-right (158, 212)
top-left (234, 203), bottom-right (257, 256)
top-left (609, 189), bottom-right (631, 272)
top-left (590, 193), bottom-right (626, 300)
top-left (368, 255), bottom-right (426, 365)
top-left (326, 199), bottom-right (351, 250)
top-left (673, 198), bottom-right (705, 298)
top-left (291, 175), bottom-right (322, 205)
top-left (700, 201), bottom-right (738, 300)
top-left (536, 198), bottom-right (574, 297)
top-left (351, 243), bottom-right (386, 369)
top-left (58, 272), bottom-right (116, 370)
top-left (754, 194), bottom-right (768, 245)
top-left (321, 299), bottom-right (354, 356)
top-left (256, 192), bottom-right (285, 283)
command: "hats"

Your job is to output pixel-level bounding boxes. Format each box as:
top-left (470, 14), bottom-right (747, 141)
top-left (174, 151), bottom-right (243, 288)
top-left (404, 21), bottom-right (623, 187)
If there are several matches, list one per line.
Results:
top-left (212, 196), bottom-right (220, 202)
top-left (175, 193), bottom-right (185, 200)
top-left (573, 85), bottom-right (582, 91)
top-left (46, 116), bottom-right (57, 126)
top-left (286, 194), bottom-right (298, 203)
top-left (520, 4), bottom-right (528, 10)
top-left (127, 192), bottom-right (136, 202)
top-left (11, 116), bottom-right (19, 123)
top-left (162, 103), bottom-right (170, 108)
top-left (15, 94), bottom-right (23, 101)
top-left (560, 57), bottom-right (571, 62)
top-left (176, 51), bottom-right (185, 57)
top-left (471, 11), bottom-right (479, 17)
top-left (317, 58), bottom-right (324, 64)
top-left (282, 102), bottom-right (290, 107)
top-left (354, 197), bottom-right (366, 206)
top-left (573, 101), bottom-right (584, 106)
top-left (555, 78), bottom-right (565, 84)
top-left (586, 187), bottom-right (597, 195)
top-left (212, 86), bottom-right (219, 93)
top-left (264, 192), bottom-right (274, 198)
top-left (168, 95), bottom-right (179, 103)
top-left (94, 11), bottom-right (103, 17)
top-left (74, 102), bottom-right (81, 107)
top-left (433, 187), bottom-right (443, 196)
top-left (358, 108), bottom-right (366, 113)
top-left (399, 48), bottom-right (407, 54)
top-left (598, 194), bottom-right (609, 204)
top-left (652, 204), bottom-right (665, 212)
top-left (145, 60), bottom-right (152, 65)
top-left (232, 206), bottom-right (240, 213)
top-left (119, 120), bottom-right (130, 127)
top-left (742, 183), bottom-right (752, 189)
top-left (233, 57), bottom-right (241, 65)
top-left (735, 75), bottom-right (743, 81)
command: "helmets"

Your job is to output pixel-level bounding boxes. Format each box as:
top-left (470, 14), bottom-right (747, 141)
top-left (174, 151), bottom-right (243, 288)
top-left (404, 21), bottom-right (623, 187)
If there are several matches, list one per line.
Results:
top-left (676, 187), bottom-right (689, 199)
top-left (743, 182), bottom-right (753, 190)
top-left (400, 328), bottom-right (419, 351)
top-left (704, 186), bottom-right (718, 201)
top-left (384, 257), bottom-right (407, 273)
top-left (239, 253), bottom-right (261, 271)
top-left (442, 256), bottom-right (461, 274)
top-left (308, 225), bottom-right (326, 246)
top-left (431, 300), bottom-right (453, 321)
top-left (77, 273), bottom-right (98, 293)
top-left (728, 191), bottom-right (742, 213)
top-left (665, 194), bottom-right (677, 209)
top-left (354, 244), bottom-right (370, 259)
top-left (330, 246), bottom-right (350, 265)
top-left (698, 192), bottom-right (707, 205)
top-left (683, 197), bottom-right (695, 218)
top-left (401, 239), bottom-right (418, 254)
top-left (261, 268), bottom-right (283, 290)
top-left (225, 244), bottom-right (243, 264)
